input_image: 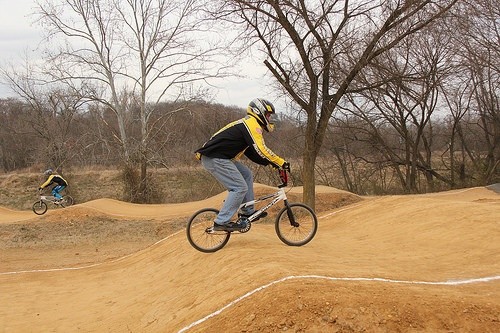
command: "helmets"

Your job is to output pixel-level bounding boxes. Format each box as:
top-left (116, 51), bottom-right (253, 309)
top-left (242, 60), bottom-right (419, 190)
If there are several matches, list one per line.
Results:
top-left (44, 170), bottom-right (53, 177)
top-left (246, 98), bottom-right (276, 132)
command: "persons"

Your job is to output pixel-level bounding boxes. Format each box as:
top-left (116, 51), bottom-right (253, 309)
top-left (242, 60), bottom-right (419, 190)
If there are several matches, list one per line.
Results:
top-left (39, 169), bottom-right (68, 202)
top-left (194, 98), bottom-right (289, 231)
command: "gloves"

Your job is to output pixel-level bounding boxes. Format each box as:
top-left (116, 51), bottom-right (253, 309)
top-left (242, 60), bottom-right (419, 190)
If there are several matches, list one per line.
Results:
top-left (37, 187), bottom-right (43, 191)
top-left (282, 162), bottom-right (290, 172)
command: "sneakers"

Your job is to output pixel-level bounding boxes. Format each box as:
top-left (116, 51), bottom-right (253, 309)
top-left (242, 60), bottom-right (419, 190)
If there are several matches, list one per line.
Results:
top-left (238, 210), bottom-right (267, 220)
top-left (214, 221), bottom-right (243, 231)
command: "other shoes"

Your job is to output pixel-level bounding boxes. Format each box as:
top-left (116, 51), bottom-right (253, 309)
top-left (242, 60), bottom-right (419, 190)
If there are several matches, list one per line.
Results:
top-left (59, 198), bottom-right (64, 204)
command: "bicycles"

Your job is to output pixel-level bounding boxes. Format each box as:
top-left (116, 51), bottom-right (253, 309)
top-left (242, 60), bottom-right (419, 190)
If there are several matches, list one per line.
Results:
top-left (186, 163), bottom-right (319, 254)
top-left (32, 190), bottom-right (74, 216)
